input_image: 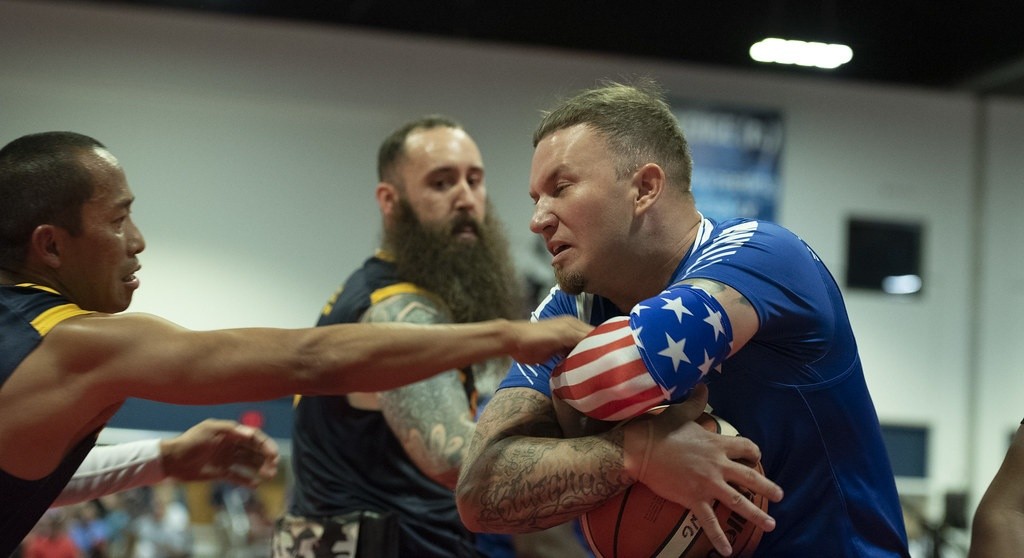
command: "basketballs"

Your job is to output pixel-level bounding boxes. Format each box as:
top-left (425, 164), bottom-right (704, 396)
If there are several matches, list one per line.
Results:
top-left (575, 405), bottom-right (767, 558)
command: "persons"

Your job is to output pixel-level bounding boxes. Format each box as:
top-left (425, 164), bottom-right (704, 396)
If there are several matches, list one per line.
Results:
top-left (287, 116), bottom-right (534, 557)
top-left (967, 418), bottom-right (1024, 557)
top-left (455, 78), bottom-right (910, 558)
top-left (0, 130), bottom-right (597, 558)
